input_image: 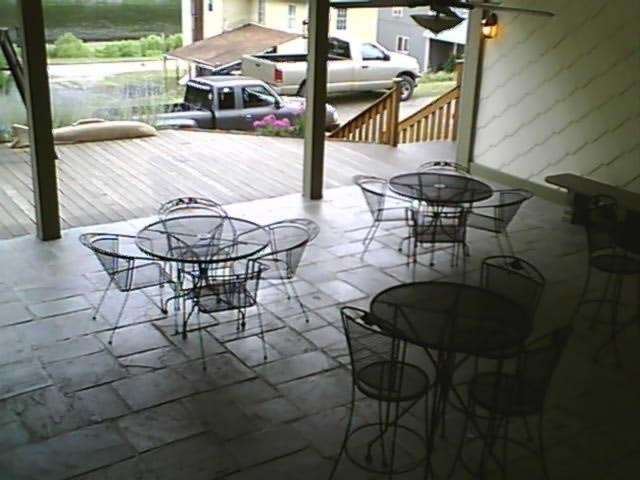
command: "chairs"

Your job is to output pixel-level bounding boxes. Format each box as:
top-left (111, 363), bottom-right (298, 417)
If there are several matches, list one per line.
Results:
top-left (79, 233), bottom-right (179, 344)
top-left (353, 161), bottom-right (535, 269)
top-left (568, 194), bottom-right (639, 367)
top-left (177, 259), bottom-right (269, 372)
top-left (232, 218), bottom-right (320, 323)
top-left (451, 327), bottom-right (572, 480)
top-left (437, 256), bottom-right (546, 414)
top-left (330, 306), bottom-right (434, 480)
top-left (158, 197), bottom-right (236, 310)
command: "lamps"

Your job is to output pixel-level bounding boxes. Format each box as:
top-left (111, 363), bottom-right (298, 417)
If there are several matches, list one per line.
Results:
top-left (407, 5), bottom-right (466, 38)
top-left (479, 10), bottom-right (499, 40)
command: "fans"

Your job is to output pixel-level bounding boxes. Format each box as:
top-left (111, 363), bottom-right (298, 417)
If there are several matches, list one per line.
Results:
top-left (330, 1), bottom-right (555, 38)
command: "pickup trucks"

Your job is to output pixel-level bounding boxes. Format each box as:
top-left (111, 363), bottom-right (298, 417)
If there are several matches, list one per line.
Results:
top-left (94, 72), bottom-right (343, 129)
top-left (240, 33), bottom-right (422, 101)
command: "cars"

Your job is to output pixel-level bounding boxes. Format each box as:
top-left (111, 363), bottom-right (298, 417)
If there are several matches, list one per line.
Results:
top-left (0, 69), bottom-right (146, 125)
